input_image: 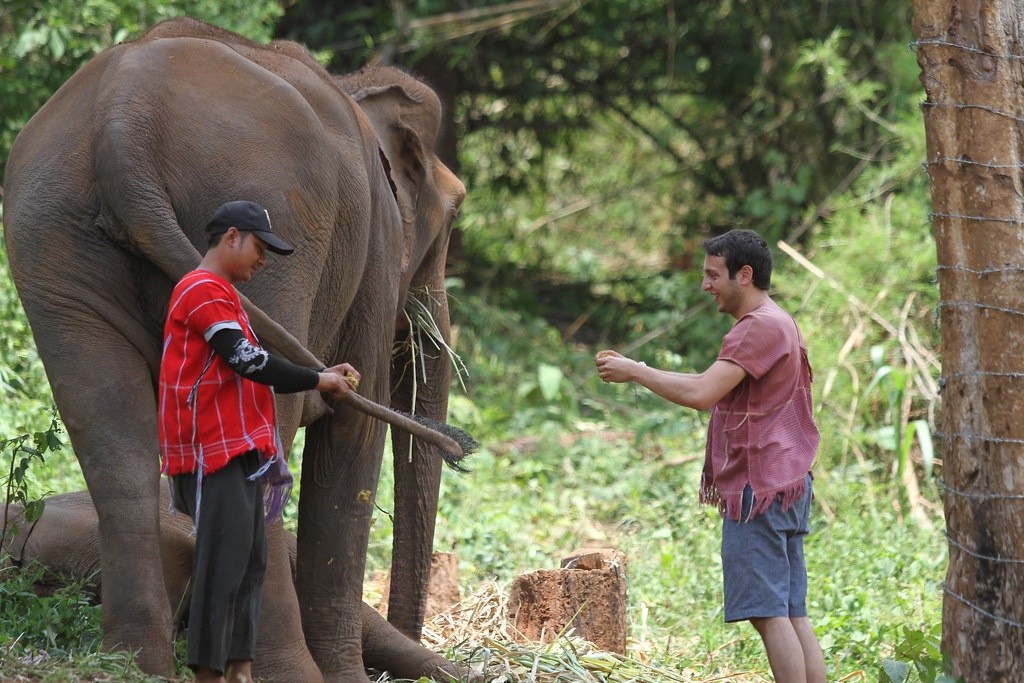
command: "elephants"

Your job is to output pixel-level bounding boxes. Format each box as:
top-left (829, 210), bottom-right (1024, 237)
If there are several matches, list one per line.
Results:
top-left (0, 18), bottom-right (479, 682)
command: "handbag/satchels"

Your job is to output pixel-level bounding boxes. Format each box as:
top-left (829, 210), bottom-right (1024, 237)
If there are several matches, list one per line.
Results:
top-left (165, 425), bottom-right (294, 530)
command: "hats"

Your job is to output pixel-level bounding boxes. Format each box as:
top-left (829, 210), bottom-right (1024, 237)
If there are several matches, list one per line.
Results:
top-left (206, 200), bottom-right (295, 256)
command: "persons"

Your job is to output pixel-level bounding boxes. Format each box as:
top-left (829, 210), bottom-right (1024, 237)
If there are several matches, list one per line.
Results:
top-left (595, 228), bottom-right (829, 683)
top-left (157, 200), bottom-right (361, 683)
